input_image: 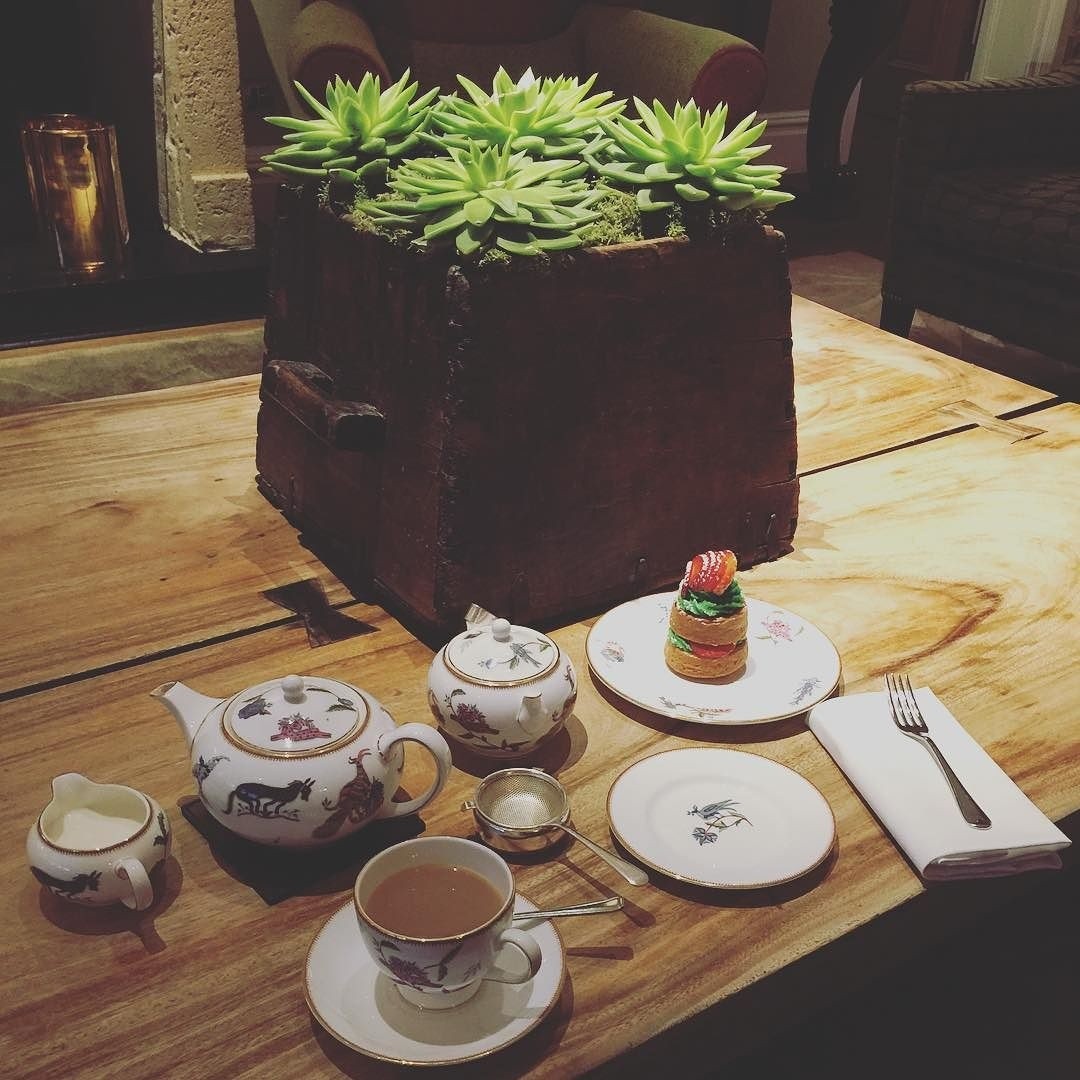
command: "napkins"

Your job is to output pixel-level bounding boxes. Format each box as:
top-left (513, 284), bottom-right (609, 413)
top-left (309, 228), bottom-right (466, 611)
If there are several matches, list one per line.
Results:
top-left (803, 687), bottom-right (1074, 881)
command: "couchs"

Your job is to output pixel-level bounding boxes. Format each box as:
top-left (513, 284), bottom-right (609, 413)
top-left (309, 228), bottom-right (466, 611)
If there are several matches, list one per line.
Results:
top-left (287, 0), bottom-right (770, 142)
top-left (879, 58), bottom-right (1080, 366)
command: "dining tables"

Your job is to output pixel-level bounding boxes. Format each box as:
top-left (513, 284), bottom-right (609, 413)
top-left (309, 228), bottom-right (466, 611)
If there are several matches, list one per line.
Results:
top-left (0, 278), bottom-right (1078, 1080)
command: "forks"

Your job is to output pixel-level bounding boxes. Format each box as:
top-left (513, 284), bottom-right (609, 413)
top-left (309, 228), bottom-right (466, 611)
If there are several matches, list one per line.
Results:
top-left (882, 670), bottom-right (992, 830)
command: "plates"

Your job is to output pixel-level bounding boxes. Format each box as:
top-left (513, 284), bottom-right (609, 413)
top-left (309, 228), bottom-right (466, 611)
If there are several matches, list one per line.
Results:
top-left (586, 591), bottom-right (844, 728)
top-left (301, 887), bottom-right (567, 1068)
top-left (605, 745), bottom-right (839, 891)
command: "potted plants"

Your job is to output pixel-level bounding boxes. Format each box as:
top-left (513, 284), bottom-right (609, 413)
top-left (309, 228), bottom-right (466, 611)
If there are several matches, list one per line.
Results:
top-left (255, 62), bottom-right (800, 633)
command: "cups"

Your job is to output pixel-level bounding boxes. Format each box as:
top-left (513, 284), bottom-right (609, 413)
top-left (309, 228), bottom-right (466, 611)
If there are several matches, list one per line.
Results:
top-left (351, 835), bottom-right (544, 1013)
top-left (20, 114), bottom-right (136, 286)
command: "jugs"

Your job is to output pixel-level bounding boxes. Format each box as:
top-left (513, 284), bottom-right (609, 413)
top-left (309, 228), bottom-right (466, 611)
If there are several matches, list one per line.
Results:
top-left (23, 768), bottom-right (170, 911)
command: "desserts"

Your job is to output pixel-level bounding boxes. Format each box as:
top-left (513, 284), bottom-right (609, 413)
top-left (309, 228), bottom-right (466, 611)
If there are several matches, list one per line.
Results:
top-left (665, 550), bottom-right (748, 680)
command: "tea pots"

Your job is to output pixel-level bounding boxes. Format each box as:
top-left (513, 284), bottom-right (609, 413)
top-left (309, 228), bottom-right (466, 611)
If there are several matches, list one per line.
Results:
top-left (149, 674), bottom-right (454, 843)
top-left (426, 607), bottom-right (578, 761)
